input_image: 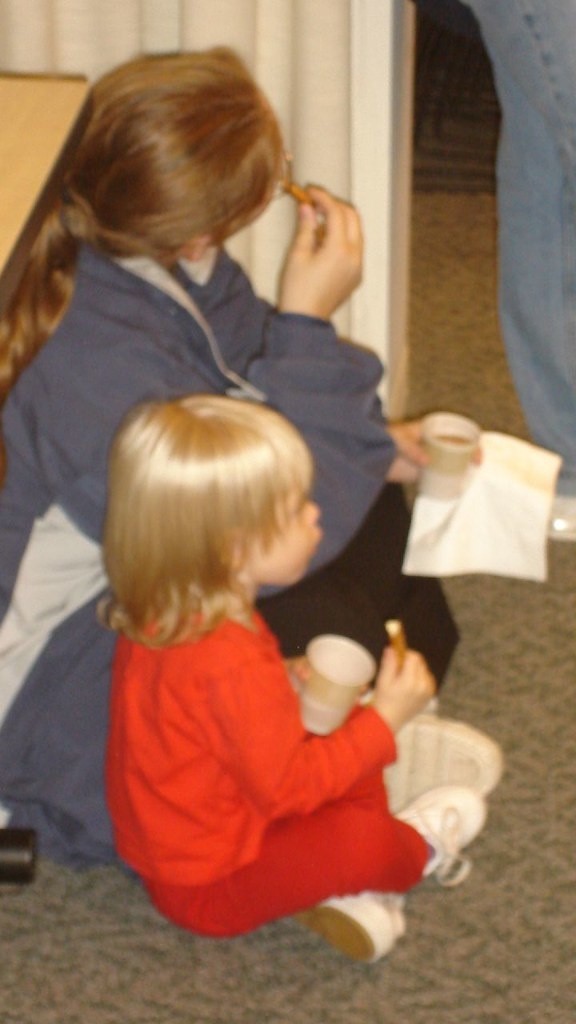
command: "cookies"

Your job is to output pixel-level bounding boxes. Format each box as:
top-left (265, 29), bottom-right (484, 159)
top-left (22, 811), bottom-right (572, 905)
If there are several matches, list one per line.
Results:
top-left (385, 618), bottom-right (407, 665)
top-left (282, 181), bottom-right (314, 206)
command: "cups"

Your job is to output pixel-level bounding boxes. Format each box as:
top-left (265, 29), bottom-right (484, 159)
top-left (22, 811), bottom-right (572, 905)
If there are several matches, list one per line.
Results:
top-left (417, 411), bottom-right (481, 499)
top-left (300, 635), bottom-right (376, 736)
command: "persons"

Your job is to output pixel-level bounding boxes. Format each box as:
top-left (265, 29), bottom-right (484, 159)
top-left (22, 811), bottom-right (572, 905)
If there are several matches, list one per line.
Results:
top-left (468, 0), bottom-right (576, 541)
top-left (95, 393), bottom-right (488, 964)
top-left (0, 48), bottom-right (484, 860)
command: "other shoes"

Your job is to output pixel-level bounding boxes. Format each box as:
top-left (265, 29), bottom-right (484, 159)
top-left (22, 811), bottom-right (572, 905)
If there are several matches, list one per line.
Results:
top-left (303, 891), bottom-right (403, 963)
top-left (548, 496), bottom-right (576, 542)
top-left (380, 698), bottom-right (507, 793)
top-left (397, 782), bottom-right (487, 887)
